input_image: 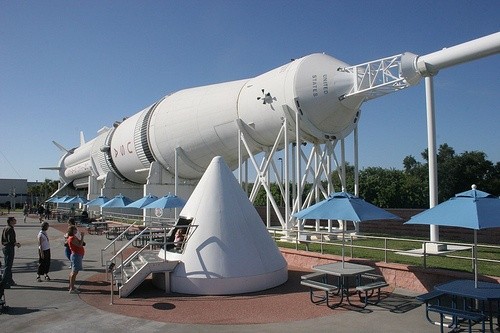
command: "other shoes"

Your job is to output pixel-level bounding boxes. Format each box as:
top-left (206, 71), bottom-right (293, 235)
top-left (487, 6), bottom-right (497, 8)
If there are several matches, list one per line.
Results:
top-left (11, 282), bottom-right (17, 286)
top-left (3, 285), bottom-right (10, 288)
top-left (69, 266), bottom-right (72, 268)
top-left (68, 287), bottom-right (81, 294)
top-left (37, 277), bottom-right (42, 282)
top-left (45, 275), bottom-right (50, 280)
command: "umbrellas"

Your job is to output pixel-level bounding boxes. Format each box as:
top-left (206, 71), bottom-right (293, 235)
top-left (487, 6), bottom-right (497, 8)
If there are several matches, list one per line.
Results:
top-left (402, 189), bottom-right (500, 289)
top-left (289, 191), bottom-right (402, 268)
top-left (44, 192), bottom-right (185, 226)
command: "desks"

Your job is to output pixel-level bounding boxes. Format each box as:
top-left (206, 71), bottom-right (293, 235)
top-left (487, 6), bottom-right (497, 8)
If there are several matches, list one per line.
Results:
top-left (311, 262), bottom-right (375, 308)
top-left (433, 279), bottom-right (500, 333)
top-left (92, 221), bottom-right (165, 248)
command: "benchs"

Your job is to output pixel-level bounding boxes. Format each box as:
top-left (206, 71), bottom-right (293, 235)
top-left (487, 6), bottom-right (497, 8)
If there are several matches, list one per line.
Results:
top-left (86, 227), bottom-right (92, 235)
top-left (301, 280), bottom-right (338, 308)
top-left (301, 271), bottom-right (325, 283)
top-left (361, 273), bottom-right (384, 280)
top-left (356, 281), bottom-right (389, 308)
top-left (426, 305), bottom-right (488, 333)
top-left (417, 290), bottom-right (447, 324)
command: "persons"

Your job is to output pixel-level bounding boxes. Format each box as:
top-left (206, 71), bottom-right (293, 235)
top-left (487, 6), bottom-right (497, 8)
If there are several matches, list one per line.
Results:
top-left (22, 200), bottom-right (50, 223)
top-left (78, 210), bottom-right (89, 225)
top-left (7, 202), bottom-right (11, 213)
top-left (69, 204), bottom-right (77, 215)
top-left (36, 222), bottom-right (51, 283)
top-left (2, 216), bottom-right (22, 289)
top-left (64, 217), bottom-right (80, 269)
top-left (63, 225), bottom-right (85, 294)
